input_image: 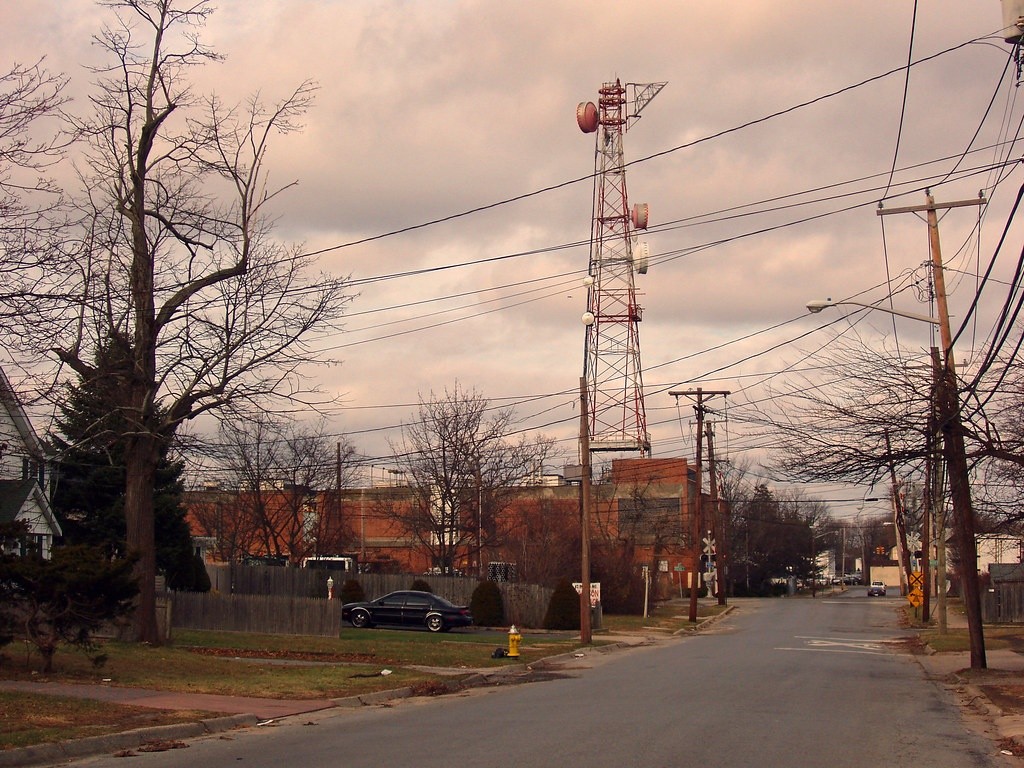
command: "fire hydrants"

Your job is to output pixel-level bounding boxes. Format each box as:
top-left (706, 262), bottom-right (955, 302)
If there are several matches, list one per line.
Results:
top-left (507, 624), bottom-right (524, 657)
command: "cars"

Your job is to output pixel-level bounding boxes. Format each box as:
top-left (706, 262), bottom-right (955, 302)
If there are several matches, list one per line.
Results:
top-left (340, 590), bottom-right (475, 634)
top-left (867, 586), bottom-right (884, 596)
top-left (870, 581), bottom-right (887, 594)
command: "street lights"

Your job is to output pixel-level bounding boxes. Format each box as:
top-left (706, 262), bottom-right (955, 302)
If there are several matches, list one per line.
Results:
top-left (804, 301), bottom-right (987, 670)
top-left (863, 498), bottom-right (905, 597)
